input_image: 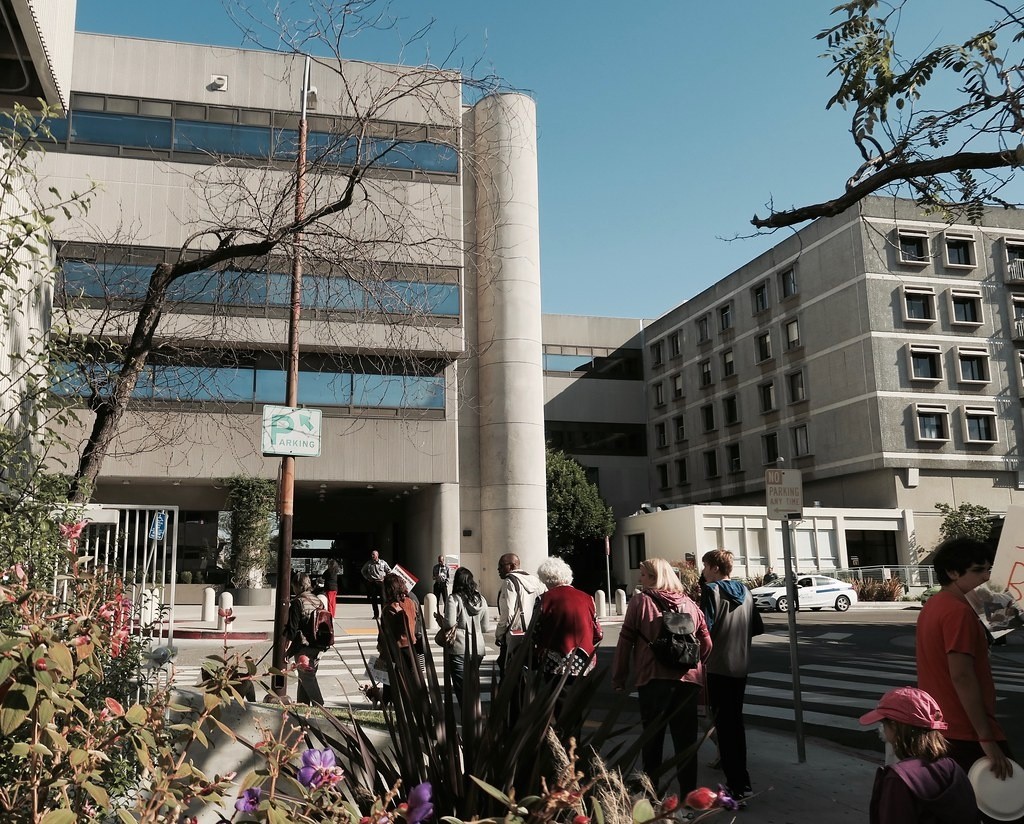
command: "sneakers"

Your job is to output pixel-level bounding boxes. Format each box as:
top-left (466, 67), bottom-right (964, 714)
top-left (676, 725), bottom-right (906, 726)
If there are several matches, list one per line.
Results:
top-left (723, 770), bottom-right (754, 806)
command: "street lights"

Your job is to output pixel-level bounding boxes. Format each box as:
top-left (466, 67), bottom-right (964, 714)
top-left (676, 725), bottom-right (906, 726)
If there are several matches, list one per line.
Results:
top-left (262, 52), bottom-right (318, 704)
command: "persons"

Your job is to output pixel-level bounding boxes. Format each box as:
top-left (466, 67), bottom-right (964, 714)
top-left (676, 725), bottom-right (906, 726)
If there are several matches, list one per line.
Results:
top-left (859, 686), bottom-right (979, 824)
top-left (284, 550), bottom-right (764, 805)
top-left (915, 538), bottom-right (1013, 781)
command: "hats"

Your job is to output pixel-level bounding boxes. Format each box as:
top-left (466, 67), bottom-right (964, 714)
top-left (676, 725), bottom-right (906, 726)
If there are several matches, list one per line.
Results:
top-left (858, 685), bottom-right (949, 731)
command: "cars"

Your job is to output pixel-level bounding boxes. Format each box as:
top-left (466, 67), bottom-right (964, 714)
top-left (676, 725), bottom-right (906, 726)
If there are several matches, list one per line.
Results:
top-left (750, 575), bottom-right (857, 613)
top-left (921, 586), bottom-right (942, 606)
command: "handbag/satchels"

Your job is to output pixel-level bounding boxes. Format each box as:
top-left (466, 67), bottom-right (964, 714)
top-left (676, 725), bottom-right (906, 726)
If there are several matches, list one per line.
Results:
top-left (434, 594), bottom-right (462, 649)
top-left (413, 611), bottom-right (429, 655)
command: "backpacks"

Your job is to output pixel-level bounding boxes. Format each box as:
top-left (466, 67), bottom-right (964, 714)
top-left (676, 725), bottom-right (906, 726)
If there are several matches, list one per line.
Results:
top-left (634, 590), bottom-right (702, 672)
top-left (293, 594), bottom-right (335, 647)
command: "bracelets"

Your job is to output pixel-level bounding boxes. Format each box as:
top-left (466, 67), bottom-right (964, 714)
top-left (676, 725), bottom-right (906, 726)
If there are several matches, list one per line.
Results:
top-left (979, 738), bottom-right (996, 742)
